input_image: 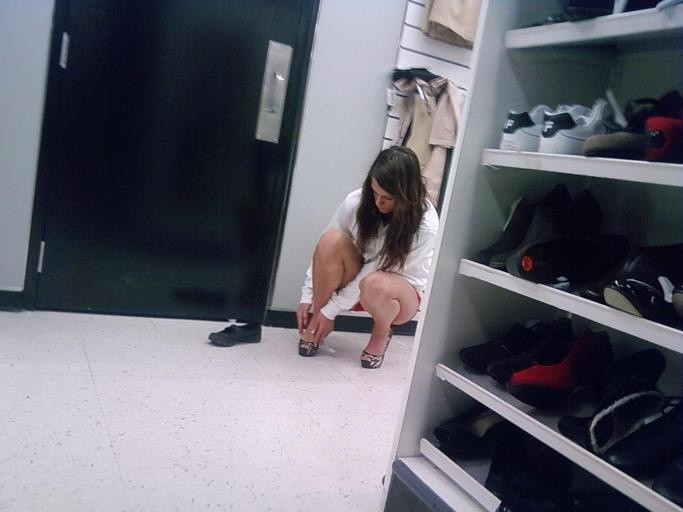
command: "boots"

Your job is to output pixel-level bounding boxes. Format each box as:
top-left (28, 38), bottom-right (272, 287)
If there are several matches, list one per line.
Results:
top-left (474, 183), bottom-right (602, 270)
top-left (559, 348), bottom-right (682, 480)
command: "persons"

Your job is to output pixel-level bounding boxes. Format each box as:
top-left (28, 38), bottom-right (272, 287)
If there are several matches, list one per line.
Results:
top-left (296, 147), bottom-right (441, 369)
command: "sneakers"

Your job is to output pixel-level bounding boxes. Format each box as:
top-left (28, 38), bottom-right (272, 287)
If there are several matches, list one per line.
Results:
top-left (500, 98), bottom-right (607, 155)
top-left (583, 109), bottom-right (683, 161)
top-left (485, 449), bottom-right (585, 512)
top-left (209, 320), bottom-right (262, 346)
top-left (506, 233), bottom-right (683, 320)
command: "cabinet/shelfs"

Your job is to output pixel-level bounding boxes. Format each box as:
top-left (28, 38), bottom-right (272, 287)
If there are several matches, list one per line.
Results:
top-left (382, 0), bottom-right (681, 511)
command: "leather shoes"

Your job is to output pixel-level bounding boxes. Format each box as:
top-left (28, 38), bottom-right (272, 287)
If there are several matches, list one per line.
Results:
top-left (434, 406), bottom-right (515, 459)
top-left (299, 333), bottom-right (320, 357)
top-left (359, 328), bottom-right (393, 368)
top-left (460, 320), bottom-right (612, 410)
